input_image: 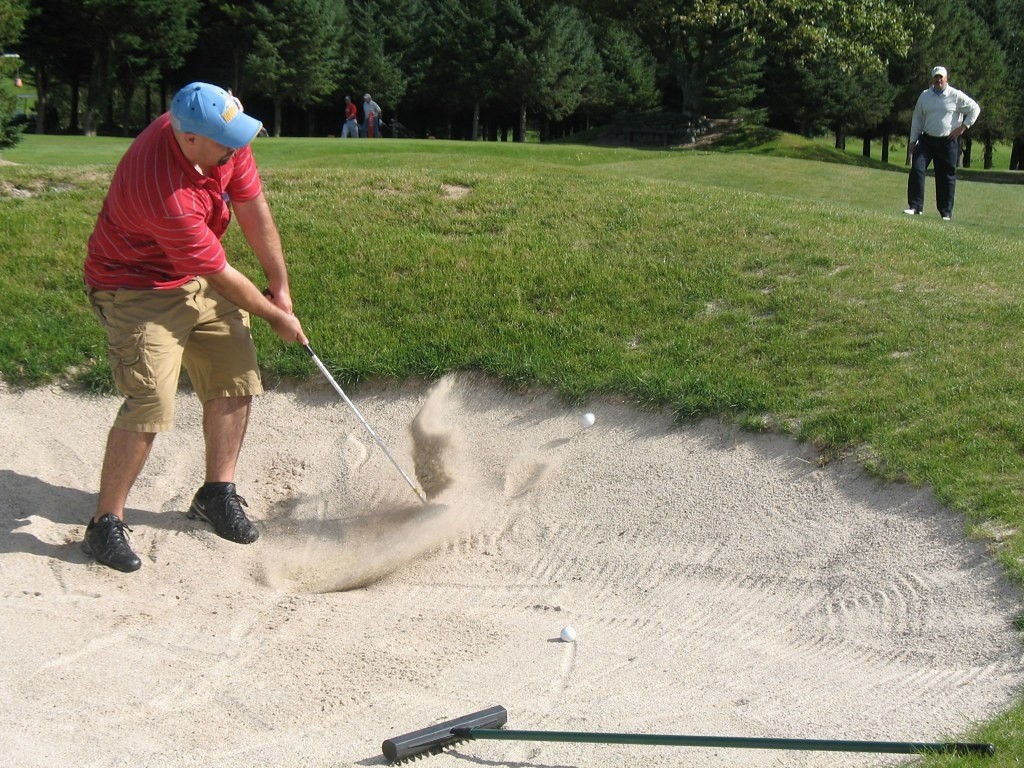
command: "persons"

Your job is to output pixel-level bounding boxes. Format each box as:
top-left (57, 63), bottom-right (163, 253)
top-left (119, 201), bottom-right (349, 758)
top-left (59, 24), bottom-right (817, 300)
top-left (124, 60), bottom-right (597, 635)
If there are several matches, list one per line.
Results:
top-left (361, 93), bottom-right (381, 137)
top-left (225, 87), bottom-right (244, 113)
top-left (903, 66), bottom-right (981, 221)
top-left (81, 81), bottom-right (308, 572)
top-left (340, 96), bottom-right (359, 138)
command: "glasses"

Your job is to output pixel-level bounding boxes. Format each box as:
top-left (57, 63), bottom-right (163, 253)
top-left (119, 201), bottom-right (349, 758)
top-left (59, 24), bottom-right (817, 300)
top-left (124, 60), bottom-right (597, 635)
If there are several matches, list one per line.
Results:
top-left (222, 192), bottom-right (230, 209)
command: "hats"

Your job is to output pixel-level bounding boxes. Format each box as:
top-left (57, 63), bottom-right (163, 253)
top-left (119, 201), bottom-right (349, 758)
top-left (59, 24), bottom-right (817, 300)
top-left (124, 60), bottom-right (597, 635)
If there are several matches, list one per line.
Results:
top-left (931, 67), bottom-right (948, 78)
top-left (170, 81), bottom-right (263, 148)
top-left (363, 93), bottom-right (371, 99)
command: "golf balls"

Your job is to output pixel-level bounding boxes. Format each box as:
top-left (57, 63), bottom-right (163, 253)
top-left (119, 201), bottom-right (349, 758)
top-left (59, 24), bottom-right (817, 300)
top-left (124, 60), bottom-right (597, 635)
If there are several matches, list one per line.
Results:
top-left (560, 626), bottom-right (576, 643)
top-left (581, 412), bottom-right (596, 427)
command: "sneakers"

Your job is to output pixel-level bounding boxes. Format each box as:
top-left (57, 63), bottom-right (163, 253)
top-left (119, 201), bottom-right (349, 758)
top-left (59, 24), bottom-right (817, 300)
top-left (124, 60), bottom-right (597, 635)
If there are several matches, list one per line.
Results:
top-left (943, 215), bottom-right (951, 220)
top-left (186, 481), bottom-right (259, 544)
top-left (902, 208), bottom-right (923, 215)
top-left (81, 513), bottom-right (141, 572)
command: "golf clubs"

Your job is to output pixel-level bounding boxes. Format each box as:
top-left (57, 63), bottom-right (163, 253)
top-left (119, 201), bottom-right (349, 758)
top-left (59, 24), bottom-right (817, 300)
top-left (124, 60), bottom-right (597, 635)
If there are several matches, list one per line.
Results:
top-left (262, 285), bottom-right (450, 512)
